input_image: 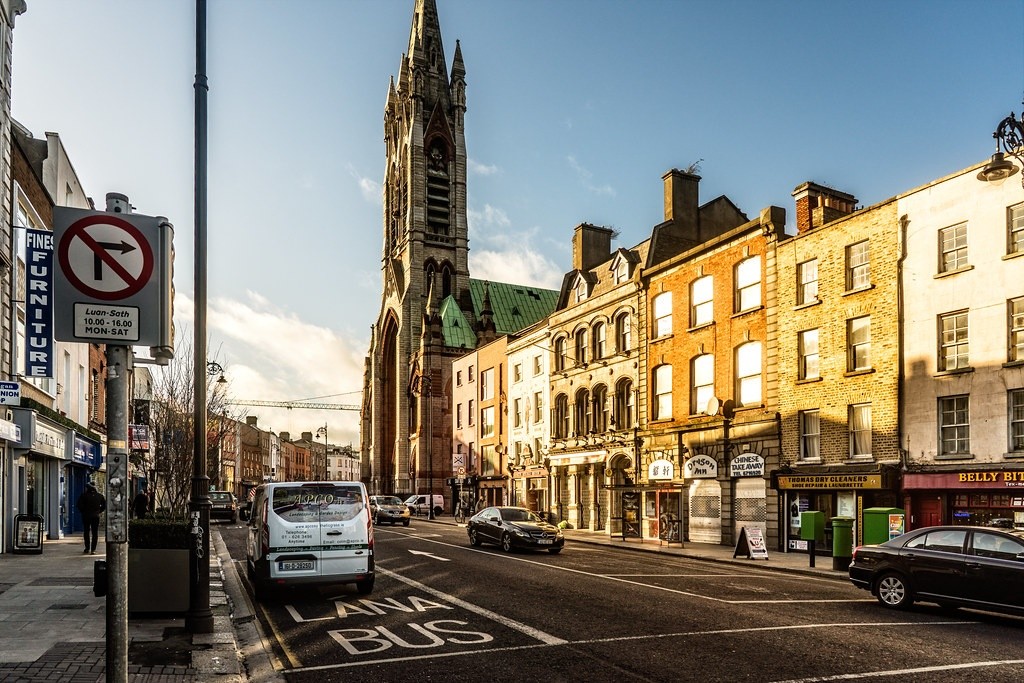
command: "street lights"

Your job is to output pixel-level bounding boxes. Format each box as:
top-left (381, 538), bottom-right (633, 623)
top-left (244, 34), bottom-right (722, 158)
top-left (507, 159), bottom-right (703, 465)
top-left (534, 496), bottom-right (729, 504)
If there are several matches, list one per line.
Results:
top-left (316, 427), bottom-right (327, 481)
top-left (270, 444), bottom-right (280, 481)
top-left (343, 446), bottom-right (353, 482)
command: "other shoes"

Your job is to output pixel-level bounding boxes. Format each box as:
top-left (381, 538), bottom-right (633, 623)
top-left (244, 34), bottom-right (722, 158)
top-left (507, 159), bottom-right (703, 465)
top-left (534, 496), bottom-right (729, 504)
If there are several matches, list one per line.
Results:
top-left (91, 551), bottom-right (95, 554)
top-left (84, 549), bottom-right (89, 553)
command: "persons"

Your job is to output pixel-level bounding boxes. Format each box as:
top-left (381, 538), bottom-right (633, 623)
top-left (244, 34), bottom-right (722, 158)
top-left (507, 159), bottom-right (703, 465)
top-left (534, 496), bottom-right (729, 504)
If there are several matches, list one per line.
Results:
top-left (78, 482), bottom-right (106, 554)
top-left (130, 490), bottom-right (149, 519)
top-left (475, 497), bottom-right (485, 513)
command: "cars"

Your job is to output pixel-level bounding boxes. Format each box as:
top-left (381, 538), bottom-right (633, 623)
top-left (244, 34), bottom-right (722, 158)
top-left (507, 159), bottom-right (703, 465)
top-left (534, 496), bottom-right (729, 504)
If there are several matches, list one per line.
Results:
top-left (466, 505), bottom-right (564, 555)
top-left (847, 522), bottom-right (1024, 617)
top-left (368, 495), bottom-right (410, 527)
top-left (222, 495), bottom-right (241, 511)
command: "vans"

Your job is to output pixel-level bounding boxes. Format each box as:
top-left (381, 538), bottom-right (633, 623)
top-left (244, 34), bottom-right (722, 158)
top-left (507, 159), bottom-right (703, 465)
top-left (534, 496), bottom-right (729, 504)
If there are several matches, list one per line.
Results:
top-left (403, 495), bottom-right (444, 517)
top-left (239, 481), bottom-right (375, 597)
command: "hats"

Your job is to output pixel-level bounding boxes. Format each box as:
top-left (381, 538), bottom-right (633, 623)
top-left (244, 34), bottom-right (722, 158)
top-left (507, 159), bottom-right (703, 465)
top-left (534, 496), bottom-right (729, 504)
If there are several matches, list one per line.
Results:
top-left (87, 482), bottom-right (96, 490)
top-left (138, 489), bottom-right (144, 494)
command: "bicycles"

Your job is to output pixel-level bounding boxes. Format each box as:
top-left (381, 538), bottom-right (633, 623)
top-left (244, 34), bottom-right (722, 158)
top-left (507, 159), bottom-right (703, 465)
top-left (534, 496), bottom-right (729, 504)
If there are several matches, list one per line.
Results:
top-left (455, 507), bottom-right (465, 524)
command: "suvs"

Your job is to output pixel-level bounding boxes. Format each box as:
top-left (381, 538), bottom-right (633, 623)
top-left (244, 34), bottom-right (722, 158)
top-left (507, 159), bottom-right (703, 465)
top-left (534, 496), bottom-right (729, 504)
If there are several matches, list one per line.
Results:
top-left (208, 491), bottom-right (236, 525)
